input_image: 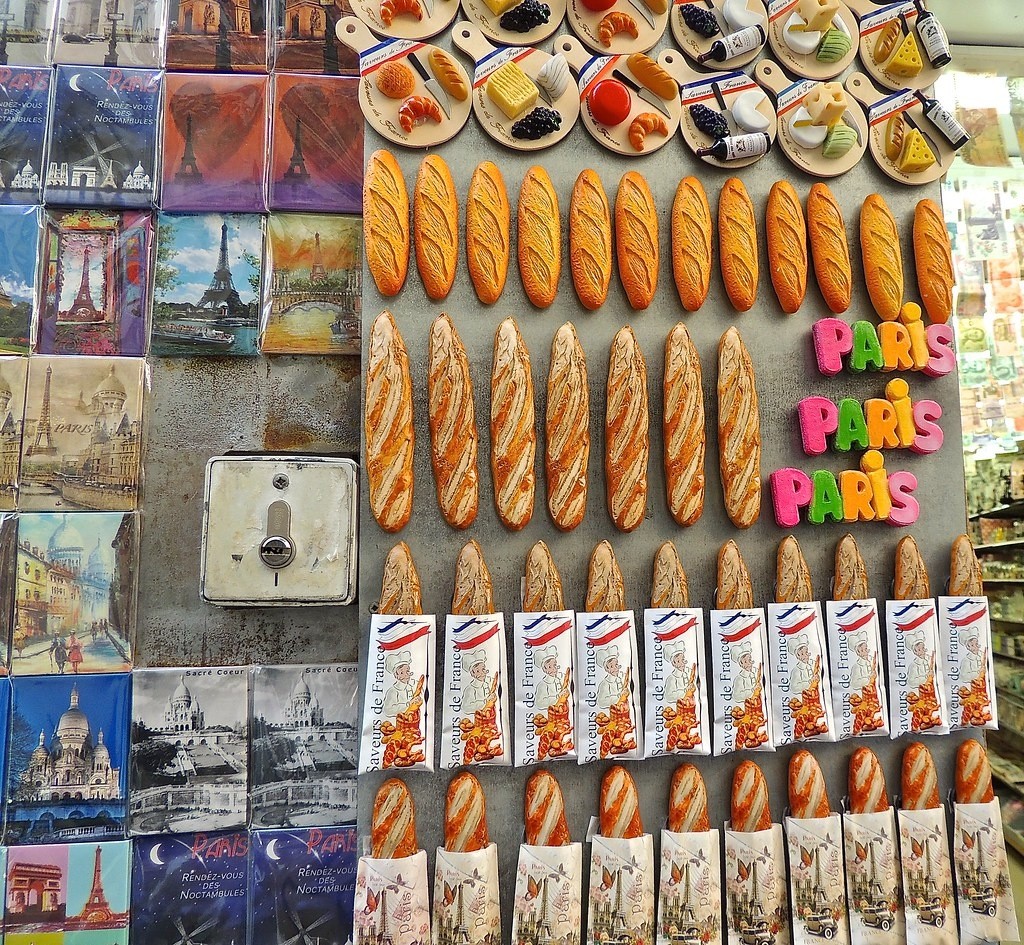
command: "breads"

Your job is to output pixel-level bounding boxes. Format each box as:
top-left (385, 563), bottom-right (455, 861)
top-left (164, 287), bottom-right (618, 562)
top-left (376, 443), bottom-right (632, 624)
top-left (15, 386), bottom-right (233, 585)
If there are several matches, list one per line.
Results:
top-left (360, 148), bottom-right (995, 861)
top-left (374, 0), bottom-right (937, 173)
